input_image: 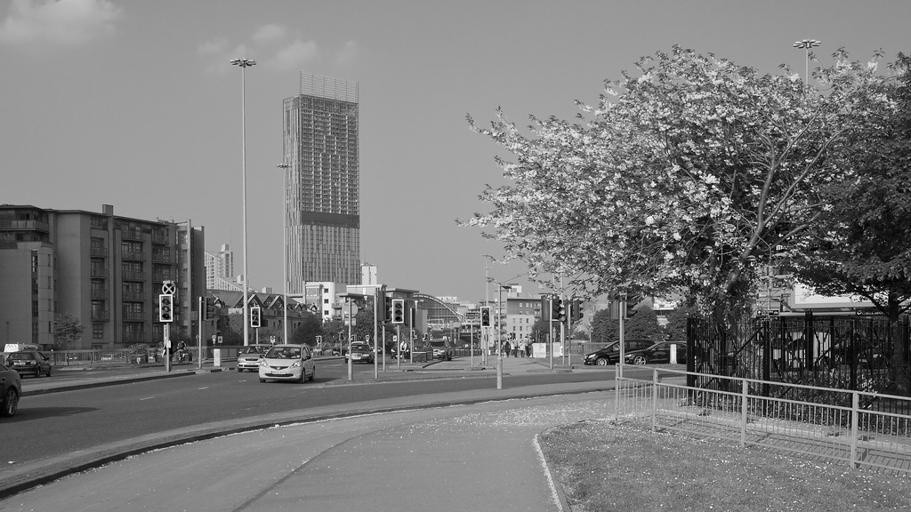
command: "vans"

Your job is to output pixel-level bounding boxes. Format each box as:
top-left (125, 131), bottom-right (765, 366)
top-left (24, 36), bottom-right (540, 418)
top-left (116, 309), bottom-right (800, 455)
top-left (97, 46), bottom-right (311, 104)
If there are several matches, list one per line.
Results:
top-left (582, 337), bottom-right (655, 365)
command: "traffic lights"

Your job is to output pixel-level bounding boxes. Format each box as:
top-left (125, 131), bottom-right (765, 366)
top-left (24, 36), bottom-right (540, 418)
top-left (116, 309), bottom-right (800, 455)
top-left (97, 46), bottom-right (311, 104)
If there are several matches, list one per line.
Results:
top-left (203, 295), bottom-right (216, 319)
top-left (479, 305), bottom-right (491, 328)
top-left (534, 293), bottom-right (586, 322)
top-left (625, 284), bottom-right (639, 322)
top-left (391, 298), bottom-right (405, 324)
top-left (159, 294), bottom-right (175, 324)
top-left (251, 307), bottom-right (261, 327)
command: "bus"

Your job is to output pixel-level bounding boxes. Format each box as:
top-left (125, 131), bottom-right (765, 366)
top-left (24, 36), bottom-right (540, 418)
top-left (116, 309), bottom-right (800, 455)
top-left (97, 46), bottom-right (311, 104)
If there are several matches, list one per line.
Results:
top-left (751, 296), bottom-right (904, 376)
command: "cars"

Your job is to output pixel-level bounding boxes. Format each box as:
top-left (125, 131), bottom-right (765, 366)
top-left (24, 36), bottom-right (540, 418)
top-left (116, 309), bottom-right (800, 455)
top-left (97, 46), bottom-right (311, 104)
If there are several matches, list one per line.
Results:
top-left (256, 344), bottom-right (317, 384)
top-left (234, 345), bottom-right (268, 371)
top-left (620, 341), bottom-right (707, 365)
top-left (854, 343), bottom-right (899, 370)
top-left (310, 335), bottom-right (455, 365)
top-left (0, 362), bottom-right (23, 416)
top-left (4, 351), bottom-right (53, 378)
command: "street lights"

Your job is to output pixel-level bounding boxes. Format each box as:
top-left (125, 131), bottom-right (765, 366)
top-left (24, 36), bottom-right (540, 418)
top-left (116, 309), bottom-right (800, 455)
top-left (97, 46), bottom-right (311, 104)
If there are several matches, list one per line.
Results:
top-left (228, 54), bottom-right (256, 347)
top-left (276, 163), bottom-right (298, 343)
top-left (496, 283), bottom-right (513, 389)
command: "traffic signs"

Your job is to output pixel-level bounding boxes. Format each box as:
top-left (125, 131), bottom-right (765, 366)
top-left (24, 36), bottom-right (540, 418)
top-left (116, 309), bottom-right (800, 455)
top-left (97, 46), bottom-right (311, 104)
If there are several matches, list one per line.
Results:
top-left (756, 309), bottom-right (781, 316)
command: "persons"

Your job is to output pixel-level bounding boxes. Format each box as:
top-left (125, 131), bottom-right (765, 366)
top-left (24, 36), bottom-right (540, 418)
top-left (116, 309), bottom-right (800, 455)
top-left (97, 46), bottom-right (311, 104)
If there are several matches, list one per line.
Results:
top-left (399, 340), bottom-right (408, 358)
top-left (162, 340), bottom-right (186, 371)
top-left (505, 340), bottom-right (511, 358)
